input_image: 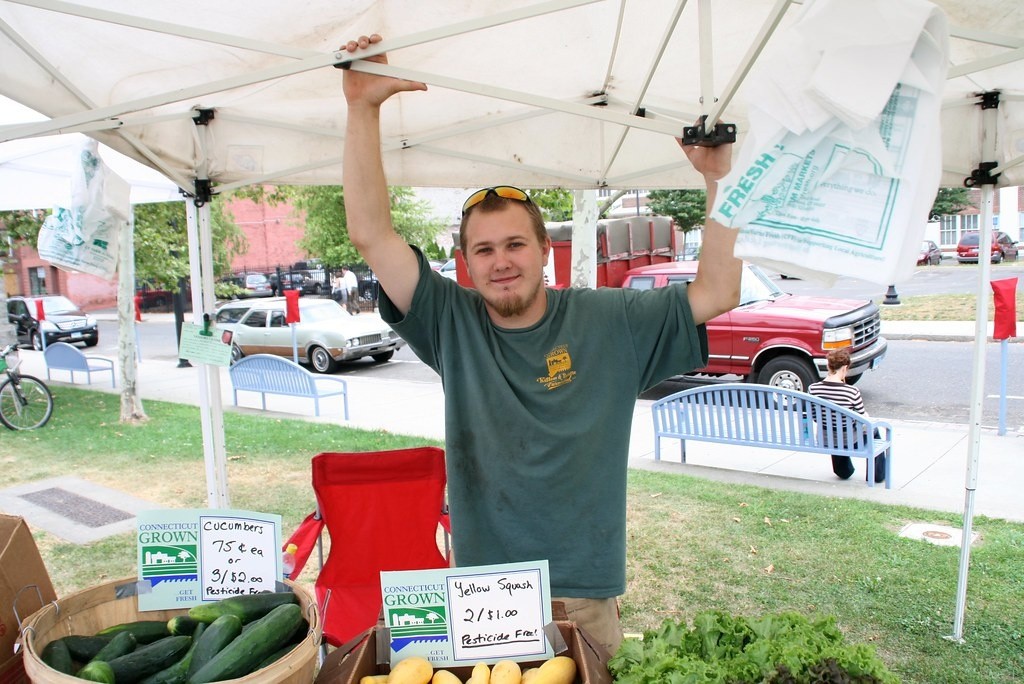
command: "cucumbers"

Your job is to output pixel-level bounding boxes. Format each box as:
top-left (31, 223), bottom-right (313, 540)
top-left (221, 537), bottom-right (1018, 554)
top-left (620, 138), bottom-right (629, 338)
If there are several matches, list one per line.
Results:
top-left (42, 590), bottom-right (304, 684)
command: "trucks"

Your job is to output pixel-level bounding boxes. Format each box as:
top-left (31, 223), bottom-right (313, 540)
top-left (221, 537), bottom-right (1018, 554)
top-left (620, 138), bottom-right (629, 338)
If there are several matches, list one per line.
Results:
top-left (451, 212), bottom-right (889, 411)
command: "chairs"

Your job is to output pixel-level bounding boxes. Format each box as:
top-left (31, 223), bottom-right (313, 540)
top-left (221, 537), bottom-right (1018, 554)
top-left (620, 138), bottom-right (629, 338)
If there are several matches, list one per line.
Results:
top-left (282, 446), bottom-right (452, 669)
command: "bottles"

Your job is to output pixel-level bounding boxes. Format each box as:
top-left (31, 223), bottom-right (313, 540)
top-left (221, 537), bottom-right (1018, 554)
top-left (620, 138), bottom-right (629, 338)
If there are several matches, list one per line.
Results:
top-left (281, 543), bottom-right (298, 580)
top-left (803, 414), bottom-right (808, 440)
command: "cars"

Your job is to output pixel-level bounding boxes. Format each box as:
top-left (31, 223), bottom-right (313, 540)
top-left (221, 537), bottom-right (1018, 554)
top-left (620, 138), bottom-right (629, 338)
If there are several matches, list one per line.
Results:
top-left (914, 240), bottom-right (943, 266)
top-left (234, 256), bottom-right (378, 302)
top-left (428, 259), bottom-right (456, 283)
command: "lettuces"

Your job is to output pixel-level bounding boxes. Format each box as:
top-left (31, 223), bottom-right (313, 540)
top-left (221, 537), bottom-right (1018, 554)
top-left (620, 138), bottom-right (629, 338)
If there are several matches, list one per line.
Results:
top-left (608, 610), bottom-right (902, 684)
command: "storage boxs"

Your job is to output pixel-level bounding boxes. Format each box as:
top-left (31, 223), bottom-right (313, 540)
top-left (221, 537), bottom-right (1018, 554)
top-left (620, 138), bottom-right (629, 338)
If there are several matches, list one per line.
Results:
top-left (0, 513), bottom-right (57, 684)
top-left (315, 601), bottom-right (611, 683)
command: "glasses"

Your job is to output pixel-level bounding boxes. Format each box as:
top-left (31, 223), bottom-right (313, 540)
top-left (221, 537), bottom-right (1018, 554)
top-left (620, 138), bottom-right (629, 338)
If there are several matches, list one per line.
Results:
top-left (461, 185), bottom-right (533, 216)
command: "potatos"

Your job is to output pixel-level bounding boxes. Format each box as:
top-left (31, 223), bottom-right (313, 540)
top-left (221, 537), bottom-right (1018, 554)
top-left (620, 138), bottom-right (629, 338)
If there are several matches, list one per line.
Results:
top-left (359, 655), bottom-right (577, 684)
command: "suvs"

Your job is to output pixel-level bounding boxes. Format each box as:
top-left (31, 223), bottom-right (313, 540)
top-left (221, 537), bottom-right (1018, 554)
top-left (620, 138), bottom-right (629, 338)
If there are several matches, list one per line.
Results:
top-left (114, 275), bottom-right (191, 308)
top-left (213, 297), bottom-right (406, 374)
top-left (7, 295), bottom-right (99, 351)
top-left (956, 230), bottom-right (1019, 264)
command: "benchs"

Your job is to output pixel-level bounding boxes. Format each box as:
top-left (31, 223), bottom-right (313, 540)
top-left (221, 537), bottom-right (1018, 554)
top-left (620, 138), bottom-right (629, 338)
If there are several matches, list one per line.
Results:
top-left (45, 342), bottom-right (115, 387)
top-left (228, 353), bottom-right (350, 418)
top-left (651, 384), bottom-right (893, 490)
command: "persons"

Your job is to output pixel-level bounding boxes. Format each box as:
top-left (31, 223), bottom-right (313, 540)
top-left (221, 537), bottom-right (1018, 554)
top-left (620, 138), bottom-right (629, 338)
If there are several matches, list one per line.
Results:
top-left (333, 266), bottom-right (360, 315)
top-left (340, 34), bottom-right (742, 653)
top-left (809, 347), bottom-right (886, 483)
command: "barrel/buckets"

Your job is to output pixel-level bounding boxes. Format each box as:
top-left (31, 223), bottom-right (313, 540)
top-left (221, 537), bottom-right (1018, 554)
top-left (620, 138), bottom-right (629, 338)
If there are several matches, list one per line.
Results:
top-left (13, 571), bottom-right (331, 684)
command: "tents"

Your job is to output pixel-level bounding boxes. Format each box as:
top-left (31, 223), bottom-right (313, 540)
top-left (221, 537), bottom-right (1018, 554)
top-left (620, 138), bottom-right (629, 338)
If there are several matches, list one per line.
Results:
top-left (0, 0), bottom-right (1024, 646)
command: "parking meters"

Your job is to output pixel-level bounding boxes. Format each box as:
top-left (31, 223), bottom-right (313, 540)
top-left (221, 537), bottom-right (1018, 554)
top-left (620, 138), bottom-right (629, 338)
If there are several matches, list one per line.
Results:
top-left (283, 289), bottom-right (301, 367)
top-left (35, 298), bottom-right (46, 355)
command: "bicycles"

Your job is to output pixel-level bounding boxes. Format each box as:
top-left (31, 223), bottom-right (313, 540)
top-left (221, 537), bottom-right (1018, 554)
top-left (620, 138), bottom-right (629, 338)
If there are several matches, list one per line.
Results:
top-left (0, 341), bottom-right (53, 432)
